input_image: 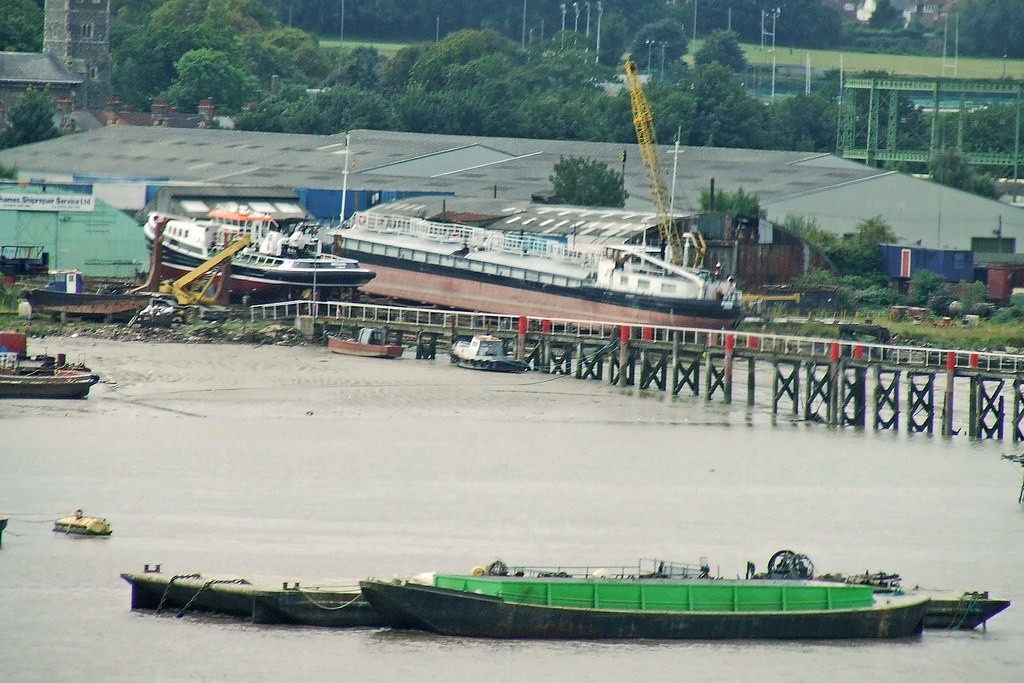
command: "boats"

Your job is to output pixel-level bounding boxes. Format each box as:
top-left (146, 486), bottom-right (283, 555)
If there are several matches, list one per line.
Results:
top-left (20, 269), bottom-right (152, 317)
top-left (120, 549), bottom-right (1012, 640)
top-left (0, 329), bottom-right (100, 399)
top-left (0, 245), bottom-right (45, 278)
top-left (141, 206), bottom-right (377, 308)
top-left (325, 210), bottom-right (744, 340)
top-left (449, 335), bottom-right (531, 373)
top-left (326, 327), bottom-right (405, 359)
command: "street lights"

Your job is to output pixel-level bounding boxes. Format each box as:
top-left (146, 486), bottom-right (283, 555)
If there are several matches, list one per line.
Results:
top-left (560, 3), bottom-right (568, 51)
top-left (584, 0), bottom-right (591, 65)
top-left (573, 2), bottom-right (581, 48)
top-left (596, 1), bottom-right (606, 68)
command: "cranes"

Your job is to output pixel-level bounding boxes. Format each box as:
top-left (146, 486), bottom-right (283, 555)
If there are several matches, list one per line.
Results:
top-left (625, 55), bottom-right (709, 273)
